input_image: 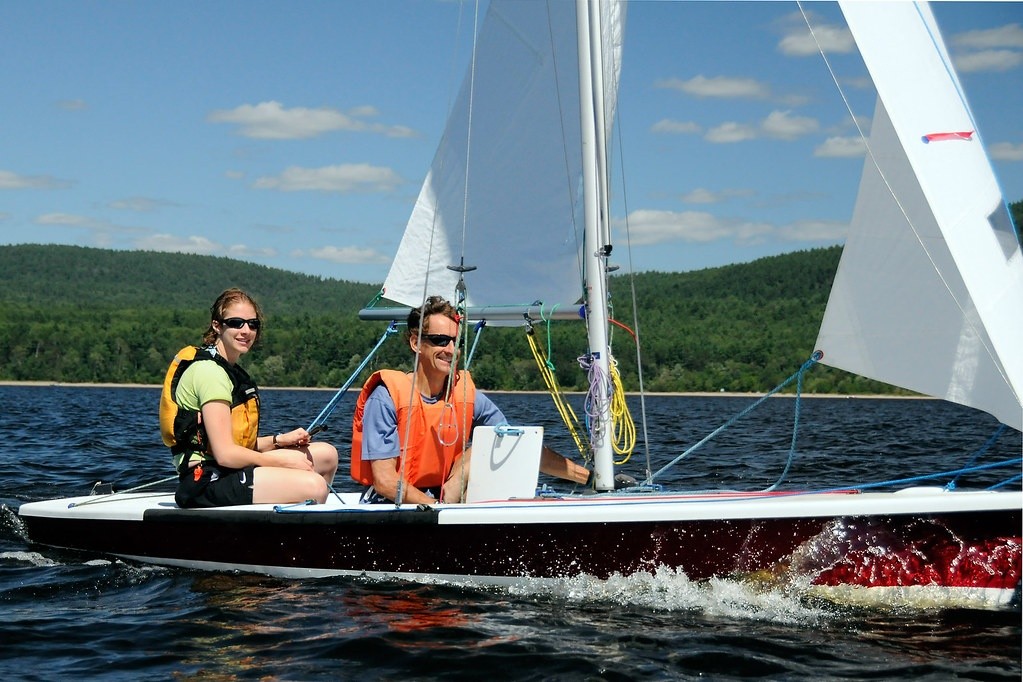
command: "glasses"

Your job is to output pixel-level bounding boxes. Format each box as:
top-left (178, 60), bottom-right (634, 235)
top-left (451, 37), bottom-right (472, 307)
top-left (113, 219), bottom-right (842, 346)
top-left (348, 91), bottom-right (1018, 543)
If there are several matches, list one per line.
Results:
top-left (419, 332), bottom-right (463, 350)
top-left (214, 316), bottom-right (262, 330)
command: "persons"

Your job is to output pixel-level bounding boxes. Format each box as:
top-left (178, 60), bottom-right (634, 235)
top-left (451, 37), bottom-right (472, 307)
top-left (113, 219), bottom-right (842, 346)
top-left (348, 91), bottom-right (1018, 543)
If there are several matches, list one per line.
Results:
top-left (159, 288), bottom-right (339, 508)
top-left (351, 296), bottom-right (636, 505)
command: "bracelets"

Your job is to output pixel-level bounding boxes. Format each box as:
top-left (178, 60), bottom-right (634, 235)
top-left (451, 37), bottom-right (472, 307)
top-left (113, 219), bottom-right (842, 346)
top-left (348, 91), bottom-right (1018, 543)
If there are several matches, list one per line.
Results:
top-left (273, 434), bottom-right (284, 449)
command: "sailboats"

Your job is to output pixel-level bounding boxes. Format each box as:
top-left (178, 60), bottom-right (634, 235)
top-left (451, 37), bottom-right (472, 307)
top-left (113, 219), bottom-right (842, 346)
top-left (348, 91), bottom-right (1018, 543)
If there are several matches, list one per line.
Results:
top-left (18, 0), bottom-right (1019, 609)
